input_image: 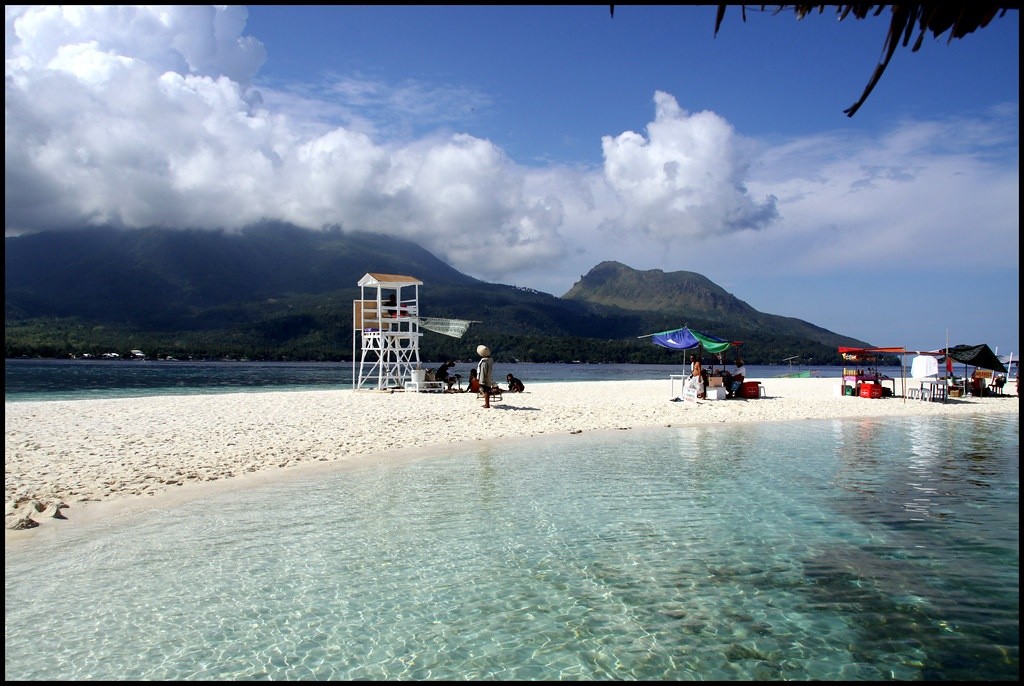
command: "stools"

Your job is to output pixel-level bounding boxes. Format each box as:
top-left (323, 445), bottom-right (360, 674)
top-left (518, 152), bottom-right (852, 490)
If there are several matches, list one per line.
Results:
top-left (907, 387), bottom-right (946, 403)
top-left (758, 386), bottom-right (766, 398)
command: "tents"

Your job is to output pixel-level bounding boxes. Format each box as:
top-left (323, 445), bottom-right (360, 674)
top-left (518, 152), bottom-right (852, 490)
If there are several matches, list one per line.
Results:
top-left (931, 344), bottom-right (1008, 397)
top-left (838, 346), bottom-right (952, 403)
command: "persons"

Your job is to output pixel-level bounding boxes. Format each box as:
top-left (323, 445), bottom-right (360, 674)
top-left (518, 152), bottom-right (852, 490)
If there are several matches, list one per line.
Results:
top-left (435, 361), bottom-right (455, 393)
top-left (465, 368), bottom-right (481, 393)
top-left (731, 359), bottom-right (745, 397)
top-left (507, 374), bottom-right (525, 393)
top-left (1016, 361), bottom-right (1019, 398)
top-left (491, 380), bottom-right (500, 393)
top-left (688, 354), bottom-right (704, 398)
top-left (384, 294), bottom-right (411, 318)
top-left (476, 345), bottom-right (494, 408)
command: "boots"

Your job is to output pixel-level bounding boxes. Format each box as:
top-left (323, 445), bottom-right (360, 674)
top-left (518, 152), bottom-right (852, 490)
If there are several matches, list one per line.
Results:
top-left (480, 393), bottom-right (490, 408)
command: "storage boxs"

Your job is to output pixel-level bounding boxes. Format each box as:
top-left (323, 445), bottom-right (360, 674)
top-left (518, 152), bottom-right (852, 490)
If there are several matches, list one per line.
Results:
top-left (859, 383), bottom-right (882, 398)
top-left (705, 385), bottom-right (727, 400)
top-left (707, 375), bottom-right (723, 387)
top-left (841, 384), bottom-right (852, 396)
top-left (743, 382), bottom-right (761, 399)
top-left (950, 389), bottom-right (960, 397)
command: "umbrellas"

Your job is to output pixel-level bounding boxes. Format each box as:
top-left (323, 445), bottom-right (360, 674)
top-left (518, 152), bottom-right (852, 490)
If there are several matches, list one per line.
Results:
top-left (637, 326), bottom-right (744, 386)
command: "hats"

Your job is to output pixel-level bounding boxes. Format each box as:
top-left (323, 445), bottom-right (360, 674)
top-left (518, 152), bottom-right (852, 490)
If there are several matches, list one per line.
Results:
top-left (477, 344), bottom-right (491, 357)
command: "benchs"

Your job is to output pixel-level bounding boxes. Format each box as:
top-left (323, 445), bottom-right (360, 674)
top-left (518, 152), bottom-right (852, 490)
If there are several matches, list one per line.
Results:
top-left (404, 381), bottom-right (444, 393)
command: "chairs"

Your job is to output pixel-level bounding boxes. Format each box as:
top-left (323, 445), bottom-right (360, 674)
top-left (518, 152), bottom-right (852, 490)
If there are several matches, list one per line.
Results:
top-left (987, 377), bottom-right (1000, 393)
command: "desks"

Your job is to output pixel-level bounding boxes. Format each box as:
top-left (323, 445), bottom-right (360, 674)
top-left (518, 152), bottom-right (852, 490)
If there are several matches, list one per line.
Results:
top-left (844, 375), bottom-right (896, 397)
top-left (670, 374), bottom-right (690, 397)
top-left (920, 379), bottom-right (948, 401)
